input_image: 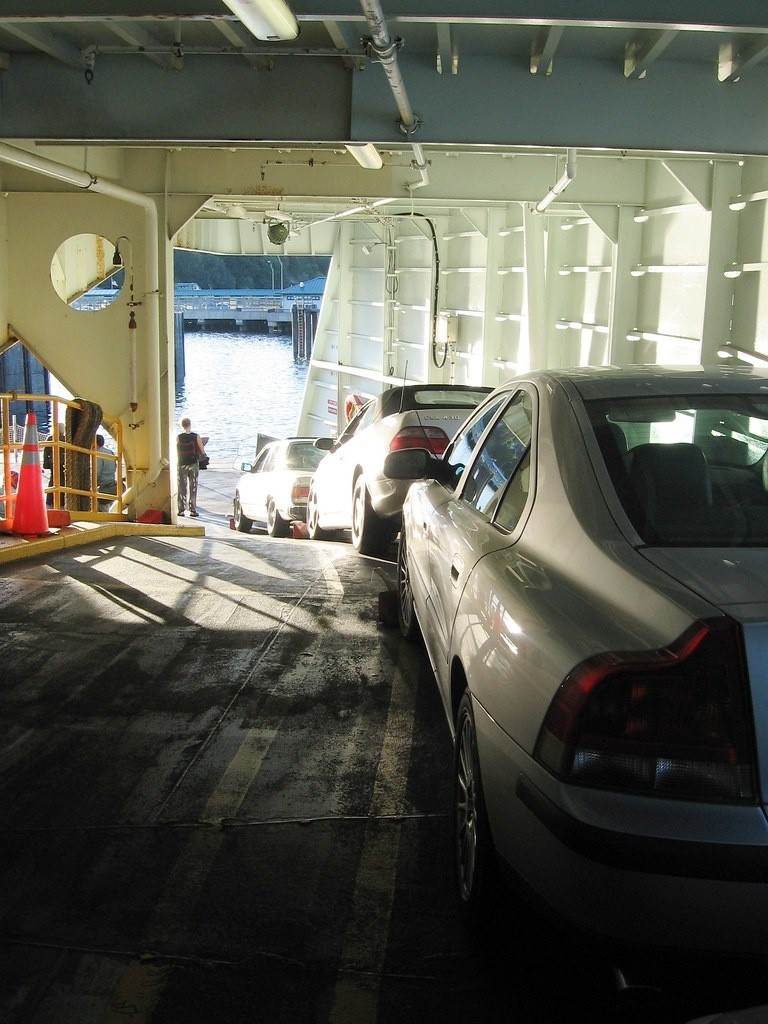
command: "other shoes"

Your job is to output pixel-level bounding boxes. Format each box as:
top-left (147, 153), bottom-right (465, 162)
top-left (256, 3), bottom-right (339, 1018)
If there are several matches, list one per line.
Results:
top-left (178, 512), bottom-right (184, 515)
top-left (190, 512), bottom-right (199, 516)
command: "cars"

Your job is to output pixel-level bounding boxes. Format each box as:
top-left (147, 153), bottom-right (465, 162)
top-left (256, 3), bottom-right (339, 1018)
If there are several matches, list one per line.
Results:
top-left (382, 363), bottom-right (768, 959)
top-left (234, 439), bottom-right (335, 538)
top-left (306, 360), bottom-right (495, 556)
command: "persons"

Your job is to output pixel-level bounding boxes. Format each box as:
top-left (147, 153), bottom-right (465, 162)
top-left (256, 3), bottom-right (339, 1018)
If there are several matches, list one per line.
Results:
top-left (177, 419), bottom-right (206, 517)
top-left (93, 435), bottom-right (116, 503)
top-left (0, 471), bottom-right (19, 519)
top-left (43, 424), bottom-right (65, 506)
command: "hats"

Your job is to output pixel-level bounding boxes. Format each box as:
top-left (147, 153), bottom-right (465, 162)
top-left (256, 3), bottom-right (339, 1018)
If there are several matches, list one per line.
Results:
top-left (10, 471), bottom-right (18, 488)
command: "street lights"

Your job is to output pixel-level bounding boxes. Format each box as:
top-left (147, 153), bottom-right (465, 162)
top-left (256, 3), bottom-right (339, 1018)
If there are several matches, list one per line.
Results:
top-left (112, 236), bottom-right (139, 522)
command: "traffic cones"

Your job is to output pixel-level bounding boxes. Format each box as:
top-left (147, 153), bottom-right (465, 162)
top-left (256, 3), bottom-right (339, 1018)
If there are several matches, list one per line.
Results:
top-left (0, 412), bottom-right (60, 538)
